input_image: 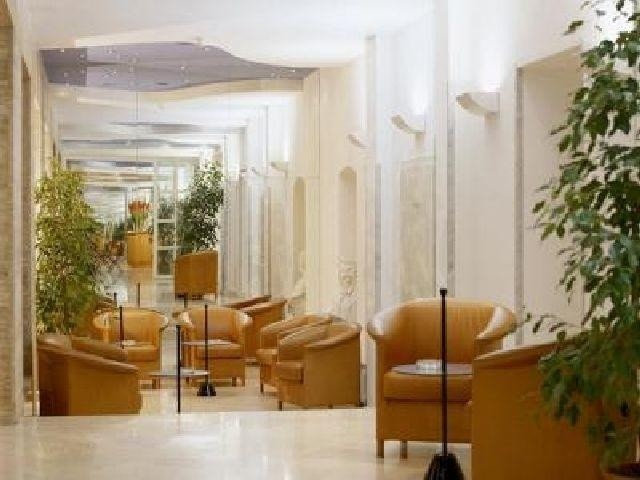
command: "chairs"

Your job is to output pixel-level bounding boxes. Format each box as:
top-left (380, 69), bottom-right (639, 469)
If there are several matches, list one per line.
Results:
top-left (180, 303), bottom-right (253, 393)
top-left (34, 328), bottom-right (145, 415)
top-left (91, 304), bottom-right (171, 389)
top-left (365, 294), bottom-right (519, 463)
top-left (219, 291), bottom-right (289, 365)
top-left (254, 311), bottom-right (335, 393)
top-left (271, 319), bottom-right (361, 412)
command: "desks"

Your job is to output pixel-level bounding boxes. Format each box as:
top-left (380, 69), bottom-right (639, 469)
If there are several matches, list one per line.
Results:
top-left (133, 367), bottom-right (211, 389)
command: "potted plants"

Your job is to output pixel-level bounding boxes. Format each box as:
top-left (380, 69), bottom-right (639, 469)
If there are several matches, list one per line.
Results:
top-left (521, 0), bottom-right (640, 480)
